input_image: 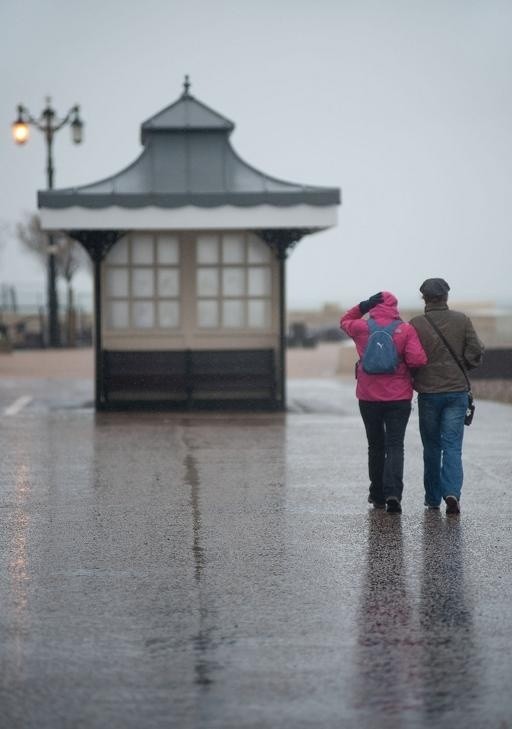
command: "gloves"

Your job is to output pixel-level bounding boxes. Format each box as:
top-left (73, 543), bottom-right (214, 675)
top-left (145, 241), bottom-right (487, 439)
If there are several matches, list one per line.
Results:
top-left (359, 292), bottom-right (384, 315)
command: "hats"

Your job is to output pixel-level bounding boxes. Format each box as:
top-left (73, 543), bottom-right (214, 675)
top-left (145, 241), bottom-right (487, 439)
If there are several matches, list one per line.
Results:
top-left (420, 278), bottom-right (450, 299)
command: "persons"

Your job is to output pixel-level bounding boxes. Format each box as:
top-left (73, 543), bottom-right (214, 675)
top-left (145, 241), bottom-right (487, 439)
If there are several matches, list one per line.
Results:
top-left (409, 278), bottom-right (484, 513)
top-left (338, 291), bottom-right (429, 516)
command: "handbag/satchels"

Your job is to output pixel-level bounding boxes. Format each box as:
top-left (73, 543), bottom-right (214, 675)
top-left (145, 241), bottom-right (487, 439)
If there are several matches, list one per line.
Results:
top-left (464, 393), bottom-right (475, 426)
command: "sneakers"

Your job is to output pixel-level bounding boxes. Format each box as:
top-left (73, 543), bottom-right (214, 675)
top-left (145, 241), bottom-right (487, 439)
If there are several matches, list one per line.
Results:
top-left (367, 494), bottom-right (402, 513)
top-left (446, 496), bottom-right (460, 514)
top-left (424, 498), bottom-right (440, 509)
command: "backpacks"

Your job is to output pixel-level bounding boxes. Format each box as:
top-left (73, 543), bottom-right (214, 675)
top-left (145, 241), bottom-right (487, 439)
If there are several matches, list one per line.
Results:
top-left (362, 319), bottom-right (402, 374)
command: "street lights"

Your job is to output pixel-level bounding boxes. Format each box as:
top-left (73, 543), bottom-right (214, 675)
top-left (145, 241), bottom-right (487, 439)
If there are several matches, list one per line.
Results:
top-left (12, 94), bottom-right (83, 346)
